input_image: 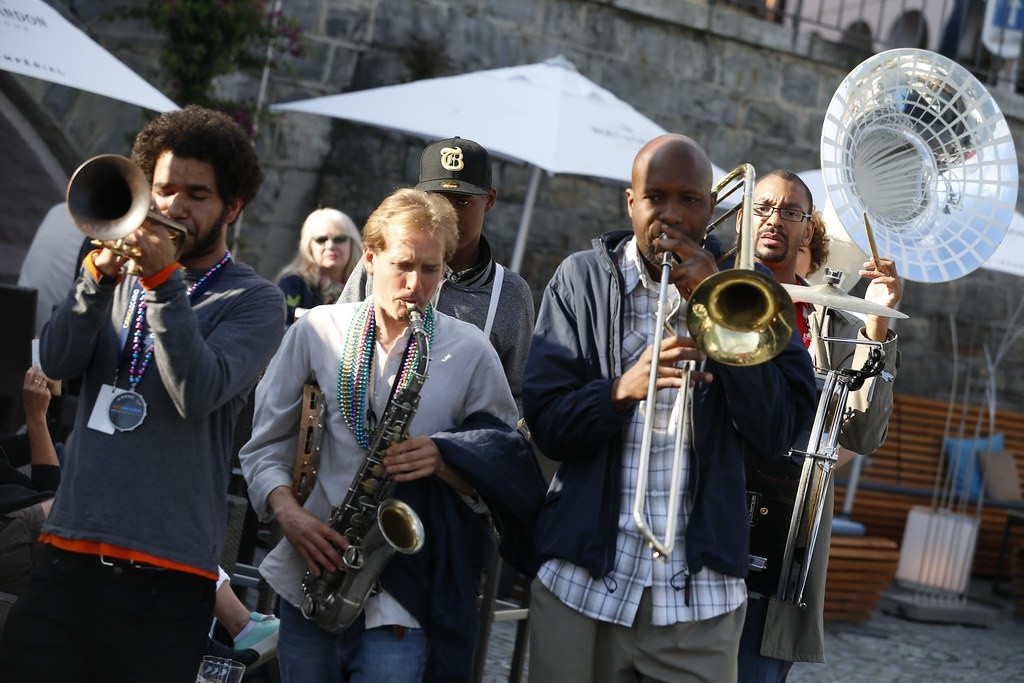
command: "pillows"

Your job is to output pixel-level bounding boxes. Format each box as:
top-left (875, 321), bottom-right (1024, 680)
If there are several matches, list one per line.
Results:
top-left (974, 448), bottom-right (1021, 501)
top-left (940, 431), bottom-right (1003, 500)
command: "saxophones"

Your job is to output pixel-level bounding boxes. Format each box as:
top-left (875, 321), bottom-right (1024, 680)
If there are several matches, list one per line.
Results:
top-left (297, 298), bottom-right (434, 636)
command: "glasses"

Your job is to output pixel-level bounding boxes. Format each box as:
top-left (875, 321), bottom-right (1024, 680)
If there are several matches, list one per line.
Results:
top-left (313, 234), bottom-right (349, 244)
top-left (753, 202), bottom-right (812, 222)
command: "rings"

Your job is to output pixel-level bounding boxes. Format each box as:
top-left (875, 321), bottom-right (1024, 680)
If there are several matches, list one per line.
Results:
top-left (41, 383), bottom-right (47, 388)
top-left (34, 379), bottom-right (41, 385)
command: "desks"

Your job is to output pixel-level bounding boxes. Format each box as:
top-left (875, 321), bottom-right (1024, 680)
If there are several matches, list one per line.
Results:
top-left (992, 509), bottom-right (1024, 598)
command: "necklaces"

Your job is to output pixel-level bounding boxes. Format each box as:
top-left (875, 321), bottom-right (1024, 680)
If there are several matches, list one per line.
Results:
top-left (337, 293), bottom-right (435, 449)
top-left (109, 247), bottom-right (230, 432)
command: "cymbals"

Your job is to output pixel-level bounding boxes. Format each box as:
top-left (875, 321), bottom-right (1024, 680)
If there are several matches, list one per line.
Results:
top-left (291, 381), bottom-right (327, 502)
top-left (780, 281), bottom-right (913, 320)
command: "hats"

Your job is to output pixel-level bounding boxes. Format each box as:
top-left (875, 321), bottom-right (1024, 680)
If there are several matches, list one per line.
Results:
top-left (413, 136), bottom-right (496, 196)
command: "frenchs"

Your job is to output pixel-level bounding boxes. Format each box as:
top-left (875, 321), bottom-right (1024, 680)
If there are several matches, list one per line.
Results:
top-left (794, 43), bottom-right (1020, 293)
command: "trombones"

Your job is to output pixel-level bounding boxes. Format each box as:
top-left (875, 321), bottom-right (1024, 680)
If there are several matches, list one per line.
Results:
top-left (630, 158), bottom-right (800, 559)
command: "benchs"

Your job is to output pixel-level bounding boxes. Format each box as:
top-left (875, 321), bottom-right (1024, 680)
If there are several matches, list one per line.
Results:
top-left (823, 392), bottom-right (1024, 622)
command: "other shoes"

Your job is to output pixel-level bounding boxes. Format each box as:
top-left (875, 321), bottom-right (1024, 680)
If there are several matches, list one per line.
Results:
top-left (205, 634), bottom-right (259, 675)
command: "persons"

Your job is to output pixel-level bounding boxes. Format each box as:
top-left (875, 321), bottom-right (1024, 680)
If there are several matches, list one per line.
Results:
top-left (246, 205), bottom-right (364, 407)
top-left (0, 201), bottom-right (282, 683)
top-left (237, 188), bottom-right (521, 683)
top-left (794, 205), bottom-right (831, 279)
top-left (525, 133), bottom-right (816, 683)
top-left (2, 104), bottom-right (287, 682)
top-left (329, 135), bottom-right (536, 683)
top-left (727, 168), bottom-right (904, 683)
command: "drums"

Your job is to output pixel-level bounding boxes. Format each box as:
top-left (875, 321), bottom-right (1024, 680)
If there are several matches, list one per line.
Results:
top-left (742, 370), bottom-right (855, 608)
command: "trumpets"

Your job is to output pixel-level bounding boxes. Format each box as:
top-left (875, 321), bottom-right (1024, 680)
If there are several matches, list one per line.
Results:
top-left (66, 153), bottom-right (189, 278)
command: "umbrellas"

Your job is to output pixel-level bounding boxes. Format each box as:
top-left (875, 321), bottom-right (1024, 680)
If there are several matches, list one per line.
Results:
top-left (269, 52), bottom-right (744, 274)
top-left (0, 0), bottom-right (181, 116)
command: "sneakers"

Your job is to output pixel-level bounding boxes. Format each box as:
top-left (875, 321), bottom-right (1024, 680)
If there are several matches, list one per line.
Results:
top-left (232, 611), bottom-right (280, 673)
